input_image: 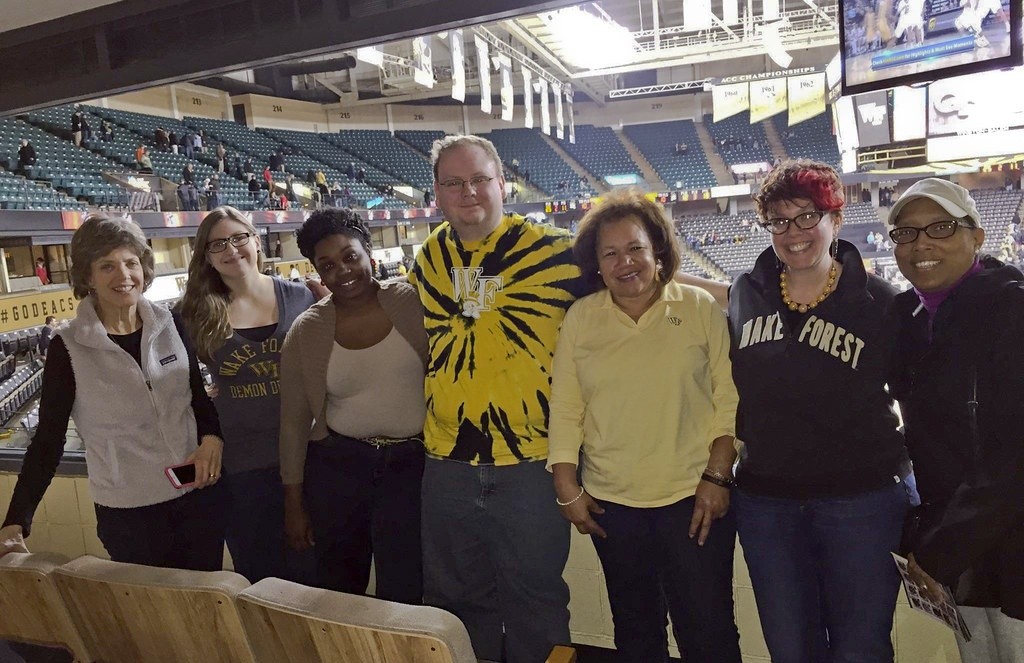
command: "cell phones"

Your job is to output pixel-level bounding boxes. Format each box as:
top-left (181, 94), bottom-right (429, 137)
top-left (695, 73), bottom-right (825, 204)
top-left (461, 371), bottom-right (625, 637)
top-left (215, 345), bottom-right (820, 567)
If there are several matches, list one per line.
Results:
top-left (164, 463), bottom-right (196, 489)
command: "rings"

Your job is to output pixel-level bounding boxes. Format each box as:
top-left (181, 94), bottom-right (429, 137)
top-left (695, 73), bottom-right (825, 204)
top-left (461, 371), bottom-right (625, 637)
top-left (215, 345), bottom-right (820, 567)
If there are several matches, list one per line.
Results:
top-left (209, 473), bottom-right (216, 478)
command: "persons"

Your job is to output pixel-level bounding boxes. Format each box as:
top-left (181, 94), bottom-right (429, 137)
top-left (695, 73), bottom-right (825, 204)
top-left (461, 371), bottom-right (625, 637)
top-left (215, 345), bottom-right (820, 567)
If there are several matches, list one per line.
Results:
top-left (0, 103), bottom-right (1024, 663)
top-left (842, 0), bottom-right (1009, 67)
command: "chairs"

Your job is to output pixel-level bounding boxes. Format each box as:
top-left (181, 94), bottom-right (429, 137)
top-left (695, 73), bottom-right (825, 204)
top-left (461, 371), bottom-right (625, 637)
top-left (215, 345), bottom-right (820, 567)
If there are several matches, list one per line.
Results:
top-left (0, 100), bottom-right (1024, 452)
top-left (0, 550), bottom-right (71, 663)
top-left (235, 575), bottom-right (578, 663)
top-left (51, 552), bottom-right (252, 663)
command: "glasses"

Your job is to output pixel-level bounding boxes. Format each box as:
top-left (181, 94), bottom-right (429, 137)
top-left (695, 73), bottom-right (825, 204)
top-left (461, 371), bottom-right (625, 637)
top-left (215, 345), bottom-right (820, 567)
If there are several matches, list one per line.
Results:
top-left (205, 232), bottom-right (252, 253)
top-left (888, 220), bottom-right (975, 244)
top-left (437, 175), bottom-right (499, 190)
top-left (757, 210), bottom-right (830, 235)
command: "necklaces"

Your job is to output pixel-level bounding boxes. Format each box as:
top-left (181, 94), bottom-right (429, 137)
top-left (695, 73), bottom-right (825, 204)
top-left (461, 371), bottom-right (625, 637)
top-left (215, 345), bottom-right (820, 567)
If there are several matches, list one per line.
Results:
top-left (779, 260), bottom-right (837, 312)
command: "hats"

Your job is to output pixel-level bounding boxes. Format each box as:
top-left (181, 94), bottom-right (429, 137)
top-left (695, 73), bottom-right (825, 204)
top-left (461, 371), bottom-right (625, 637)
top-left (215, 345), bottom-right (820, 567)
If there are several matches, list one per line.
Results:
top-left (887, 177), bottom-right (981, 226)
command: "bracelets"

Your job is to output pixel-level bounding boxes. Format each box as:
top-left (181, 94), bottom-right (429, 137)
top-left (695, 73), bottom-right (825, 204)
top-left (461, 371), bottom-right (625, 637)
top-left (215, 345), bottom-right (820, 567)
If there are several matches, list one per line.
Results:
top-left (700, 474), bottom-right (731, 488)
top-left (556, 486), bottom-right (584, 506)
top-left (706, 468), bottom-right (730, 480)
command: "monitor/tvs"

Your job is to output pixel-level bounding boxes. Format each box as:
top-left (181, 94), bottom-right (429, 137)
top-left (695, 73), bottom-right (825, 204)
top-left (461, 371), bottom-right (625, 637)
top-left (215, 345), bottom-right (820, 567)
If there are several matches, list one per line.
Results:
top-left (838, 0), bottom-right (1024, 96)
